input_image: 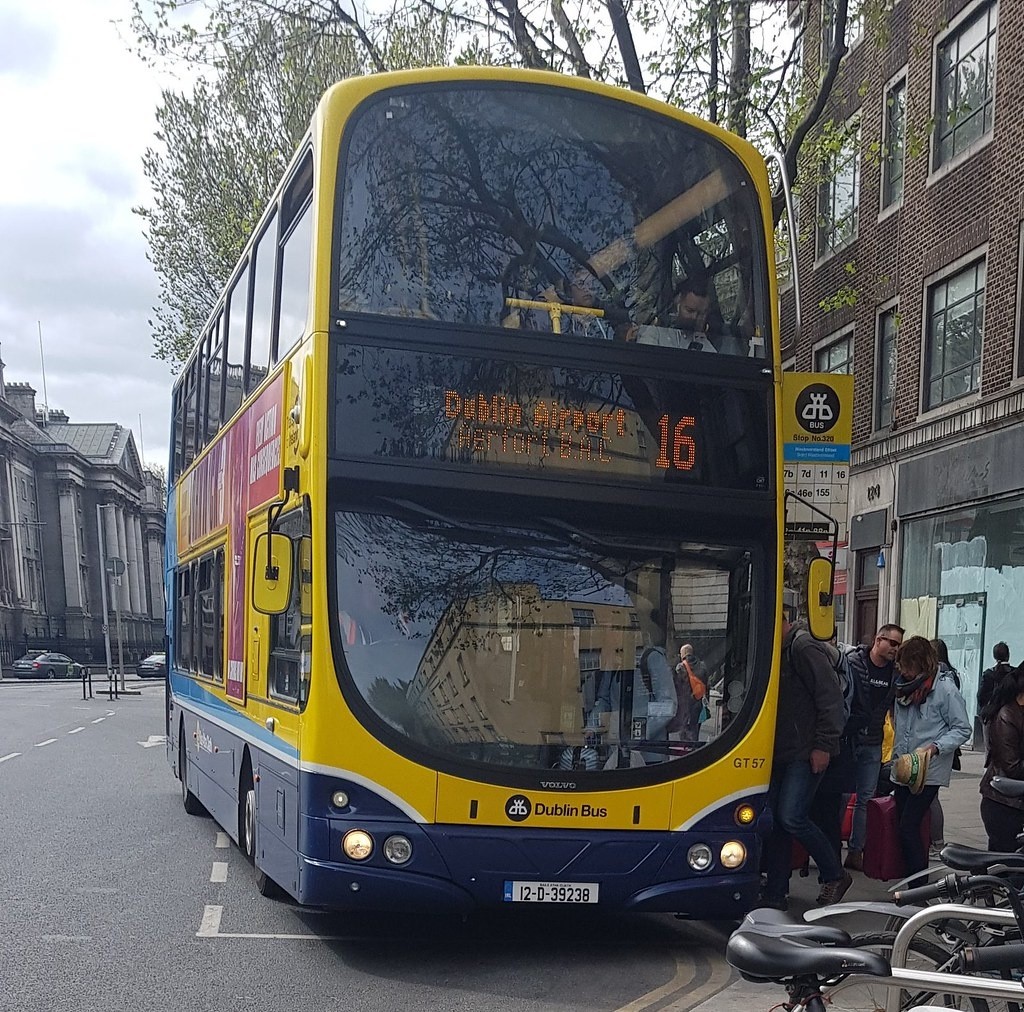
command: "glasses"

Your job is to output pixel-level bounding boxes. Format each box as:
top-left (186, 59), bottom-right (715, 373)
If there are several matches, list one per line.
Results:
top-left (881, 636), bottom-right (902, 648)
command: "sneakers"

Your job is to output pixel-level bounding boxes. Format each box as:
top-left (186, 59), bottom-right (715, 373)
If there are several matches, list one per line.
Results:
top-left (813, 867), bottom-right (855, 909)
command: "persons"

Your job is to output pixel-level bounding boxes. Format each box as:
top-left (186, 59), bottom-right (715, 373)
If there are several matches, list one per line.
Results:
top-left (758, 611), bottom-right (974, 914)
top-left (977, 640), bottom-right (1024, 853)
top-left (582, 638), bottom-right (678, 766)
top-left (338, 569), bottom-right (422, 648)
top-left (674, 643), bottom-right (710, 751)
top-left (554, 261), bottom-right (749, 356)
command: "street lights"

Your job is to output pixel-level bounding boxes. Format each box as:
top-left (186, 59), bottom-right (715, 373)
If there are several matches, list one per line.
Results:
top-left (96, 504), bottom-right (119, 679)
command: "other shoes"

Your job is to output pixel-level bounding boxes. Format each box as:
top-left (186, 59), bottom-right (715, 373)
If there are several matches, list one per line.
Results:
top-left (844, 848), bottom-right (864, 872)
top-left (928, 842), bottom-right (942, 861)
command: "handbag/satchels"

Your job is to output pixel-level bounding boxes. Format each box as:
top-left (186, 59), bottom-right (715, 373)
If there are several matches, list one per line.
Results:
top-left (683, 660), bottom-right (706, 699)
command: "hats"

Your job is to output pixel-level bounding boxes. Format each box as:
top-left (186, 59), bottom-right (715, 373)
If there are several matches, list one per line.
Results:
top-left (895, 748), bottom-right (931, 794)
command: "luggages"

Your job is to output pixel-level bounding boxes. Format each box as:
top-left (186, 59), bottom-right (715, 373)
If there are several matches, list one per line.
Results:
top-left (864, 787), bottom-right (931, 883)
top-left (789, 835), bottom-right (810, 878)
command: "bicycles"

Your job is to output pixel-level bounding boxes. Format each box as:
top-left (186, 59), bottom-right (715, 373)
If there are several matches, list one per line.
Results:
top-left (723, 774), bottom-right (1024, 1011)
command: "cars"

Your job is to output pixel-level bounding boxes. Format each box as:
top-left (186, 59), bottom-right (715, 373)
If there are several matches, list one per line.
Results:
top-left (11, 649), bottom-right (89, 680)
top-left (135, 651), bottom-right (165, 679)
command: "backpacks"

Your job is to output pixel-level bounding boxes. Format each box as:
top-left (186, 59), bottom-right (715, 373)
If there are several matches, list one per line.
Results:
top-left (788, 629), bottom-right (854, 729)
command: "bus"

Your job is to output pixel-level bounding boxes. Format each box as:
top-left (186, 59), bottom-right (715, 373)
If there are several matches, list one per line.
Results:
top-left (162, 64), bottom-right (839, 921)
